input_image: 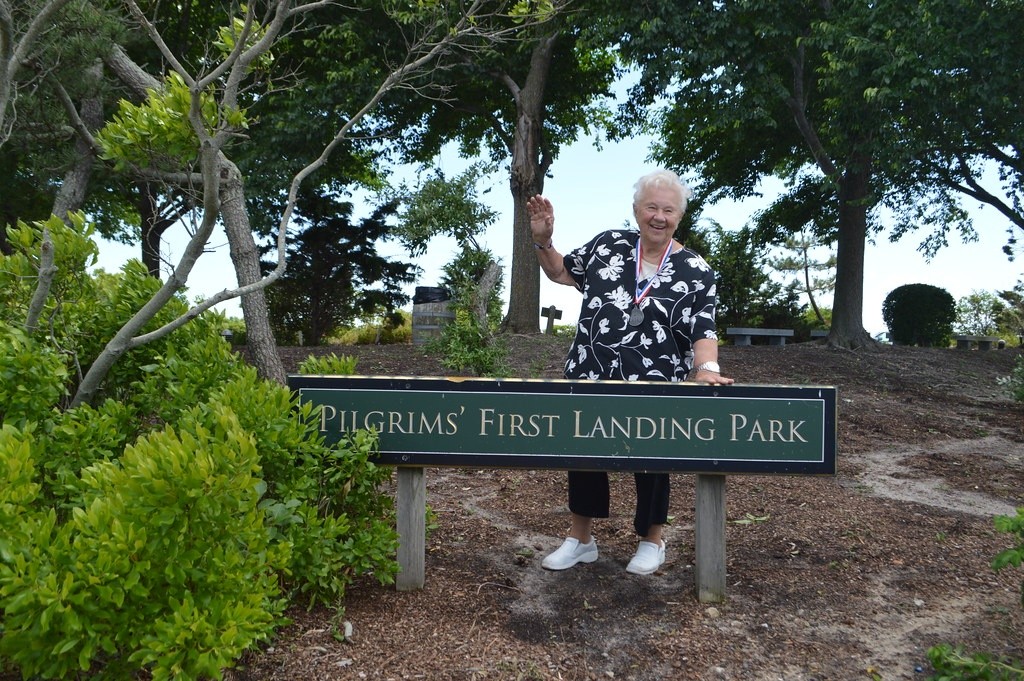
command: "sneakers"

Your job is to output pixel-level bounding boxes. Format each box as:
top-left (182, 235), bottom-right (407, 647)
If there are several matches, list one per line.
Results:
top-left (541, 537), bottom-right (599, 570)
top-left (626, 539), bottom-right (665, 575)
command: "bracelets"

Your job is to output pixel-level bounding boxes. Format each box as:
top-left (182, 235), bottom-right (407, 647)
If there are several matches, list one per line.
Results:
top-left (533, 238), bottom-right (553, 251)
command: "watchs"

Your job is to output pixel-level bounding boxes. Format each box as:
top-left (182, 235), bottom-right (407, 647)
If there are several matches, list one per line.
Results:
top-left (693, 361), bottom-right (720, 374)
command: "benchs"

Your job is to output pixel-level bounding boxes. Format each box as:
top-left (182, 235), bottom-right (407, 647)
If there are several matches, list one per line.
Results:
top-left (952, 335), bottom-right (1000, 351)
top-left (811, 329), bottom-right (829, 339)
top-left (727, 327), bottom-right (794, 345)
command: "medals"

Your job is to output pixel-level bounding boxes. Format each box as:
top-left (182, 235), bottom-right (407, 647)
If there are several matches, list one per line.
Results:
top-left (628, 236), bottom-right (676, 327)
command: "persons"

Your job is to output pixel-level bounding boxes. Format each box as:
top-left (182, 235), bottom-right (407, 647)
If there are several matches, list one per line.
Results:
top-left (527, 169), bottom-right (735, 576)
